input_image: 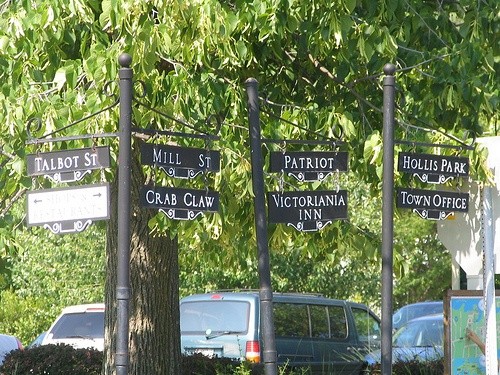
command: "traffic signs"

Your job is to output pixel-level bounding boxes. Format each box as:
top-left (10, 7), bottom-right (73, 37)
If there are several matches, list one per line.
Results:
top-left (140, 143), bottom-right (223, 220)
top-left (396, 151), bottom-right (471, 222)
top-left (22, 146), bottom-right (112, 232)
top-left (267, 151), bottom-right (350, 233)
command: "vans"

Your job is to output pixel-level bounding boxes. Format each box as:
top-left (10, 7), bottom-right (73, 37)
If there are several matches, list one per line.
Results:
top-left (42, 303), bottom-right (108, 350)
top-left (180, 290), bottom-right (382, 374)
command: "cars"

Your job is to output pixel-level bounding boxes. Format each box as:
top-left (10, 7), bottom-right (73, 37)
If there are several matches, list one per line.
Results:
top-left (363, 313), bottom-right (444, 366)
top-left (0, 333), bottom-right (23, 366)
top-left (392, 300), bottom-right (443, 328)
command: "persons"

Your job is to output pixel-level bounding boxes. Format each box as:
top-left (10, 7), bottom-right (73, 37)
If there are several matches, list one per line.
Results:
top-left (466, 327), bottom-right (500, 370)
